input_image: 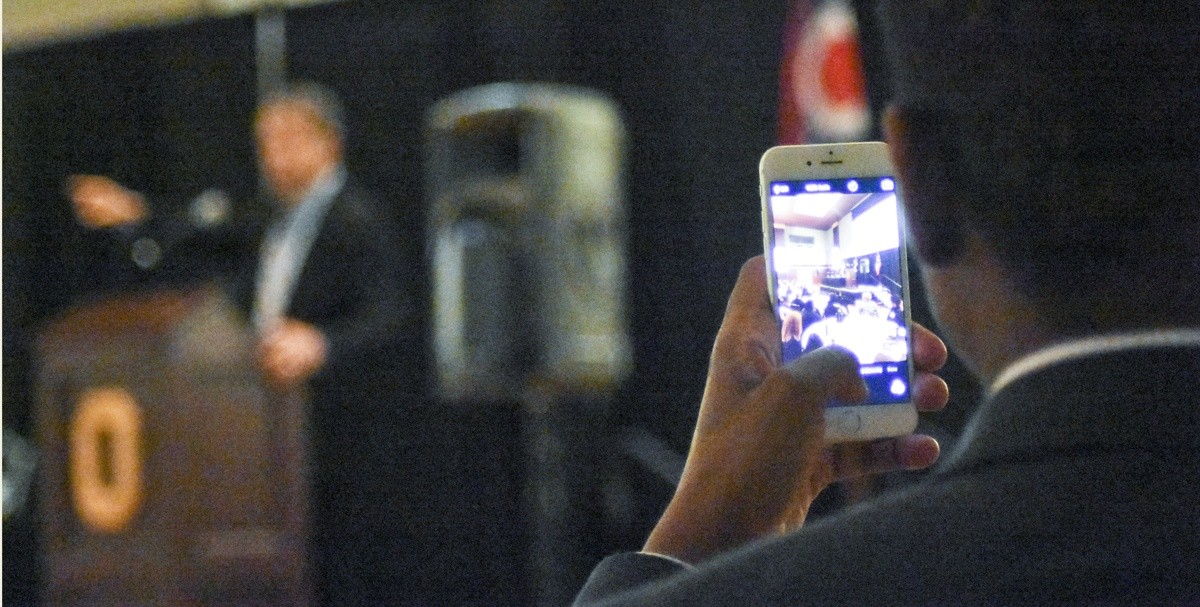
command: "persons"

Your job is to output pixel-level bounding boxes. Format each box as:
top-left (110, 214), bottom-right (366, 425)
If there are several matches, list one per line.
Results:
top-left (537, 0), bottom-right (1198, 607)
top-left (774, 254), bottom-right (909, 373)
top-left (57, 73), bottom-right (425, 569)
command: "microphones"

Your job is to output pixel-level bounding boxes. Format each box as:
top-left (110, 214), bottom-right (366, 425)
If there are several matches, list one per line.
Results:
top-left (113, 188), bottom-right (228, 286)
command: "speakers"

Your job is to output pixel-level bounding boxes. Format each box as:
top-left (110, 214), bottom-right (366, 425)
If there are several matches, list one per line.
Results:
top-left (426, 82), bottom-right (633, 401)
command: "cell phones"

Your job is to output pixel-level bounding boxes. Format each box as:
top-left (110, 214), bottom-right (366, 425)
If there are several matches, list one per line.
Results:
top-left (756, 141), bottom-right (918, 449)
